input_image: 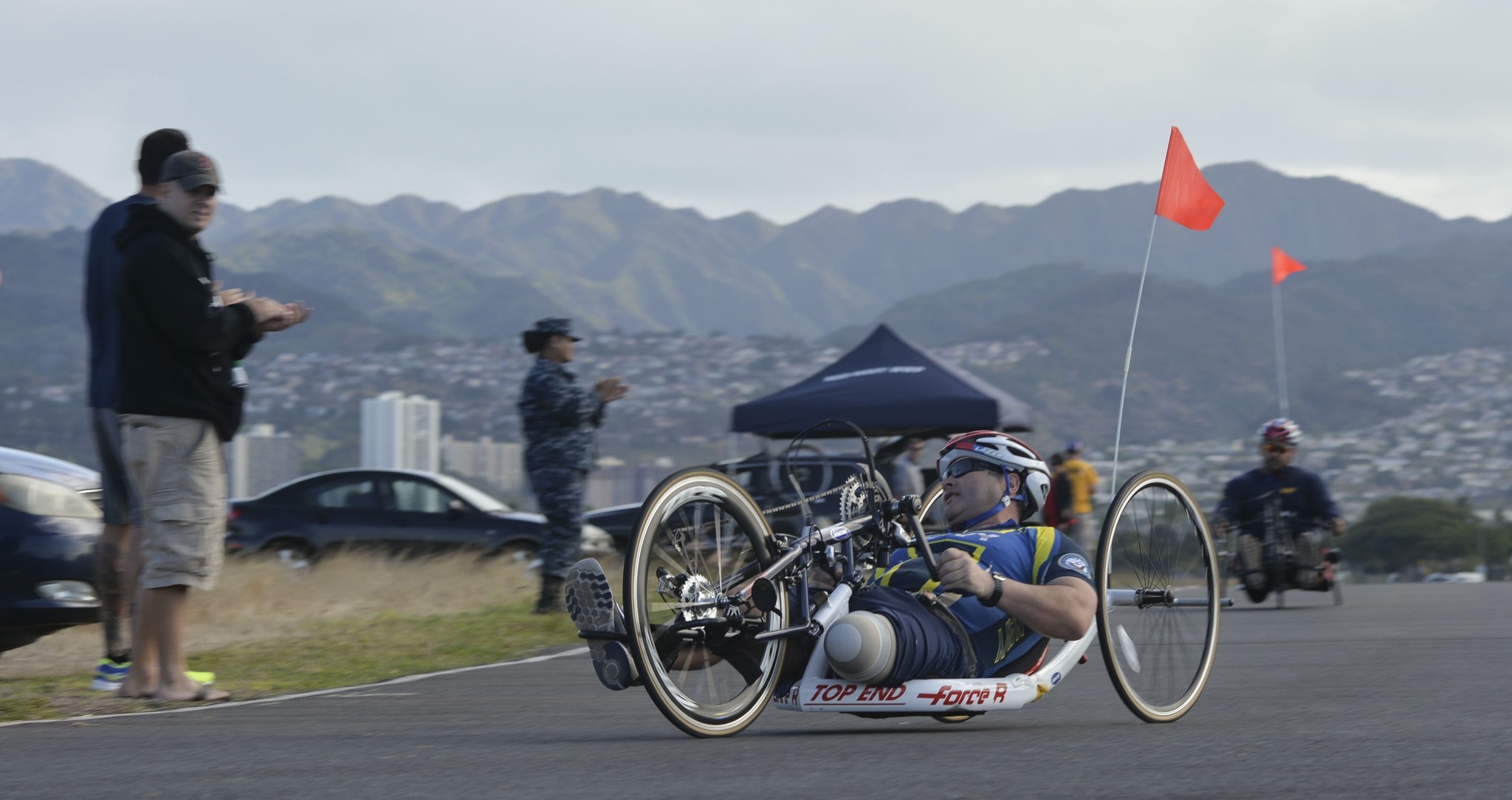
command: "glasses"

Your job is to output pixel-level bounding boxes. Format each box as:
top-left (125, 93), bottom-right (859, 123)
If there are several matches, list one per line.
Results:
top-left (1263, 445), bottom-right (1288, 454)
top-left (909, 446), bottom-right (926, 450)
top-left (941, 458), bottom-right (1004, 480)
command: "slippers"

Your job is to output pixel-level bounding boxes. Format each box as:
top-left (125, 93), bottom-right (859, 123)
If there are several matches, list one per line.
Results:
top-left (119, 692), bottom-right (158, 700)
top-left (159, 684), bottom-right (232, 703)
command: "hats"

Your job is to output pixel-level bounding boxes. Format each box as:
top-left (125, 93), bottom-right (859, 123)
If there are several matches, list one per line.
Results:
top-left (160, 150), bottom-right (221, 193)
top-left (533, 317), bottom-right (584, 340)
top-left (1067, 441), bottom-right (1085, 450)
top-left (144, 129), bottom-right (188, 157)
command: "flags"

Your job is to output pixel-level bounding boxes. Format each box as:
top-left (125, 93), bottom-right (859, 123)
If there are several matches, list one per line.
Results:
top-left (1154, 127), bottom-right (1225, 231)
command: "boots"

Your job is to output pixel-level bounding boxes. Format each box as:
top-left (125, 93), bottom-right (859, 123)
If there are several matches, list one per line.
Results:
top-left (533, 578), bottom-right (574, 615)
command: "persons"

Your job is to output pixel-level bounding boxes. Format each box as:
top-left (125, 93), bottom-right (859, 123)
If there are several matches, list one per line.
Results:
top-left (564, 429), bottom-right (1098, 692)
top-left (517, 316), bottom-right (631, 617)
top-left (82, 126), bottom-right (316, 707)
top-left (893, 436), bottom-right (927, 498)
top-left (1045, 442), bottom-right (1101, 570)
top-left (1209, 419), bottom-right (1348, 591)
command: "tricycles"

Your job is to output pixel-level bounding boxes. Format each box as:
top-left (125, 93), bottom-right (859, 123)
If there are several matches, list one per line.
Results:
top-left (576, 417), bottom-right (1237, 739)
top-left (1223, 480), bottom-right (1344, 610)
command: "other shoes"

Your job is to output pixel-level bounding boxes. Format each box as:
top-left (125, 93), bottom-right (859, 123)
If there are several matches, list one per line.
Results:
top-left (1298, 532), bottom-right (1316, 586)
top-left (565, 558), bottom-right (652, 691)
top-left (1239, 534), bottom-right (1269, 602)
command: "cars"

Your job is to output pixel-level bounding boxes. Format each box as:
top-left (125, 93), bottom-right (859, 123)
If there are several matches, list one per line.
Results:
top-left (225, 464), bottom-right (618, 590)
top-left (576, 446), bottom-right (938, 563)
top-left (0, 445), bottom-right (105, 628)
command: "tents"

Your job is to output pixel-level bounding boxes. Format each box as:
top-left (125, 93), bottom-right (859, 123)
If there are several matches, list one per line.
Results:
top-left (725, 319), bottom-right (1034, 575)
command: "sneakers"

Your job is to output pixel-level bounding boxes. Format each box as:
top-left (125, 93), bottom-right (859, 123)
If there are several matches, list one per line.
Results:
top-left (90, 656), bottom-right (217, 691)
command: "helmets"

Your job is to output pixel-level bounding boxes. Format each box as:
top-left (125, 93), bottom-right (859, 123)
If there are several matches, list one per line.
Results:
top-left (936, 431), bottom-right (1051, 522)
top-left (1258, 417), bottom-right (1305, 449)
